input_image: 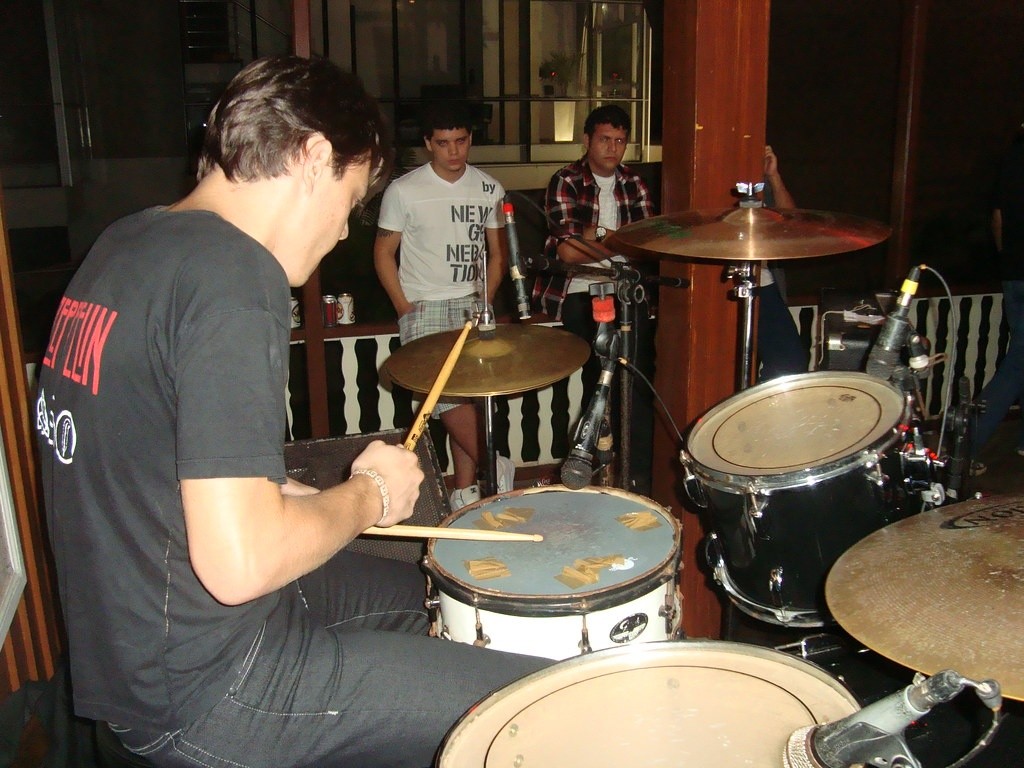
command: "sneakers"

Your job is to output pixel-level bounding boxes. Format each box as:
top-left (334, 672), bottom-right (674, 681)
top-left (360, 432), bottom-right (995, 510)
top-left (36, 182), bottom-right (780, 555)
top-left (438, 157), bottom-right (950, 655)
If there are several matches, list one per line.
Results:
top-left (448, 483), bottom-right (480, 512)
top-left (597, 415), bottom-right (614, 451)
top-left (496, 454), bottom-right (516, 494)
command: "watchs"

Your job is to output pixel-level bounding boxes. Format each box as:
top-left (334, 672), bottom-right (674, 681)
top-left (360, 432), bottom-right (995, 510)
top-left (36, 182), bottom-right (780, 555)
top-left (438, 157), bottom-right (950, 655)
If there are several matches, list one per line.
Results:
top-left (595, 226), bottom-right (607, 242)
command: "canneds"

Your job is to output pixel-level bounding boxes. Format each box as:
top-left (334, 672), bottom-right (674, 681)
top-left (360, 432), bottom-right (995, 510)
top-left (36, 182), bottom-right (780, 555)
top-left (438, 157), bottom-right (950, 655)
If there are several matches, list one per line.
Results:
top-left (337, 292), bottom-right (355, 325)
top-left (322, 294), bottom-right (337, 327)
top-left (291, 297), bottom-right (300, 329)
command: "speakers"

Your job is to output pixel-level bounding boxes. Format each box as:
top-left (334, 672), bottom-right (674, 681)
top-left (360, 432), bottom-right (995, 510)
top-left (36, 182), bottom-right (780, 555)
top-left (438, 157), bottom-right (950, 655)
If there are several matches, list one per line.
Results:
top-left (284, 423), bottom-right (453, 564)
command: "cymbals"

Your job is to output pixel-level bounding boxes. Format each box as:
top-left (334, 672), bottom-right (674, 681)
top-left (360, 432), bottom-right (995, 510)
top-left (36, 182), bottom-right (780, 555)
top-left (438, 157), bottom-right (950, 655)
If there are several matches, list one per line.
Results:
top-left (820, 490), bottom-right (1024, 705)
top-left (611, 210), bottom-right (895, 263)
top-left (382, 324), bottom-right (593, 397)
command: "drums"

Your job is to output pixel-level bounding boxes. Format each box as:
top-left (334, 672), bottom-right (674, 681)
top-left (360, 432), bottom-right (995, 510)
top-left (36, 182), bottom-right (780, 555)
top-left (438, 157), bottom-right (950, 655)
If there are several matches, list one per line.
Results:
top-left (419, 481), bottom-right (687, 664)
top-left (677, 370), bottom-right (931, 630)
top-left (432, 642), bottom-right (872, 768)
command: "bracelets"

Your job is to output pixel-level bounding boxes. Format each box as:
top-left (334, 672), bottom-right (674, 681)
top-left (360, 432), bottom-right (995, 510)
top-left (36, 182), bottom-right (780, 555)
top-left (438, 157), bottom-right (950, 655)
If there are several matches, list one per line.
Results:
top-left (349, 470), bottom-right (389, 525)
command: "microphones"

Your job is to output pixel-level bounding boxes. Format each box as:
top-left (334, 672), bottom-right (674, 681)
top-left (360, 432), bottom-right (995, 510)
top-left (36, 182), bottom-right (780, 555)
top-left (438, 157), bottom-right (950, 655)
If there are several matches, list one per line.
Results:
top-left (560, 359), bottom-right (616, 490)
top-left (501, 195), bottom-right (532, 321)
top-left (782, 669), bottom-right (966, 768)
top-left (866, 267), bottom-right (921, 379)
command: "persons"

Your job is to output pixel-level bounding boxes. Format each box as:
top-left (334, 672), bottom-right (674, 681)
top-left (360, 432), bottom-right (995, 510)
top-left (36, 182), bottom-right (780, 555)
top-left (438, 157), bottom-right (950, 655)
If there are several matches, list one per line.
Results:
top-left (33, 57), bottom-right (561, 768)
top-left (374, 99), bottom-right (508, 512)
top-left (947, 132), bottom-right (1024, 474)
top-left (754, 140), bottom-right (810, 384)
top-left (530, 105), bottom-right (657, 495)
